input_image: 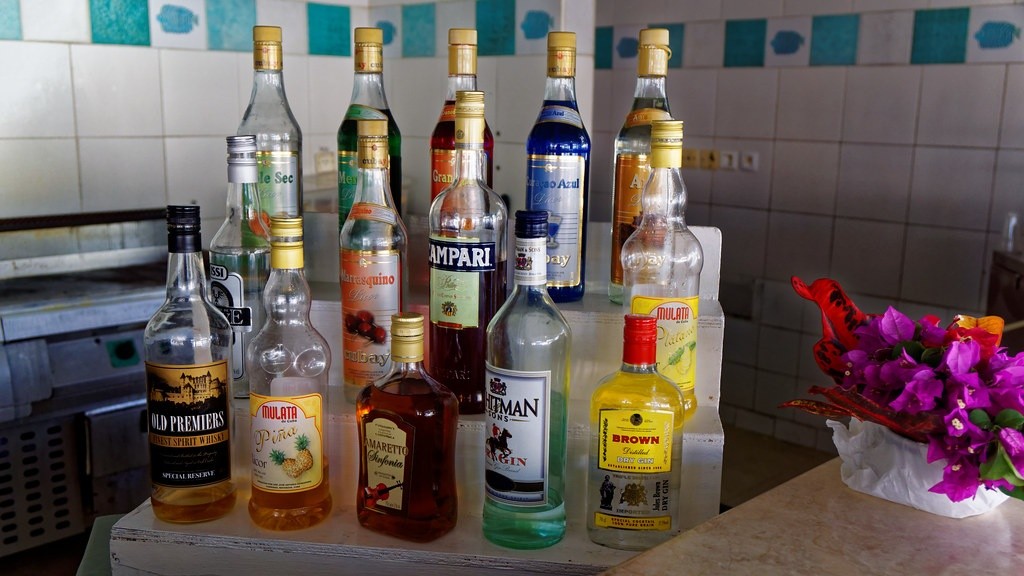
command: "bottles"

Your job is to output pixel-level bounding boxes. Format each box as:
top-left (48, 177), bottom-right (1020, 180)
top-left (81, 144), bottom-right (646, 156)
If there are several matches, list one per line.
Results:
top-left (207, 136), bottom-right (274, 402)
top-left (587, 316), bottom-right (687, 555)
top-left (527, 29), bottom-right (592, 303)
top-left (485, 212), bottom-right (572, 549)
top-left (143, 204), bottom-right (237, 524)
top-left (621, 120), bottom-right (705, 429)
top-left (336, 26), bottom-right (402, 230)
top-left (429, 28), bottom-right (495, 207)
top-left (246, 216), bottom-right (331, 533)
top-left (356, 313), bottom-right (461, 544)
top-left (339, 117), bottom-right (411, 406)
top-left (608, 28), bottom-right (674, 308)
top-left (235, 25), bottom-right (304, 271)
top-left (430, 90), bottom-right (511, 415)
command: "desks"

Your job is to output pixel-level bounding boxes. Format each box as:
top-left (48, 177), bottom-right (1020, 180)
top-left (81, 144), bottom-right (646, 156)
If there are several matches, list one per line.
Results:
top-left (596, 453), bottom-right (1023, 576)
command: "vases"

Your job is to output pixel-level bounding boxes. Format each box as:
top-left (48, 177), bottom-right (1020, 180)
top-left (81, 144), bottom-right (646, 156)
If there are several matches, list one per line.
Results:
top-left (826, 417), bottom-right (1011, 519)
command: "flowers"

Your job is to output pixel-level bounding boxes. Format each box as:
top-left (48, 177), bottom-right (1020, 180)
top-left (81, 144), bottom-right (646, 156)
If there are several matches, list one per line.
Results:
top-left (777, 277), bottom-right (1024, 502)
